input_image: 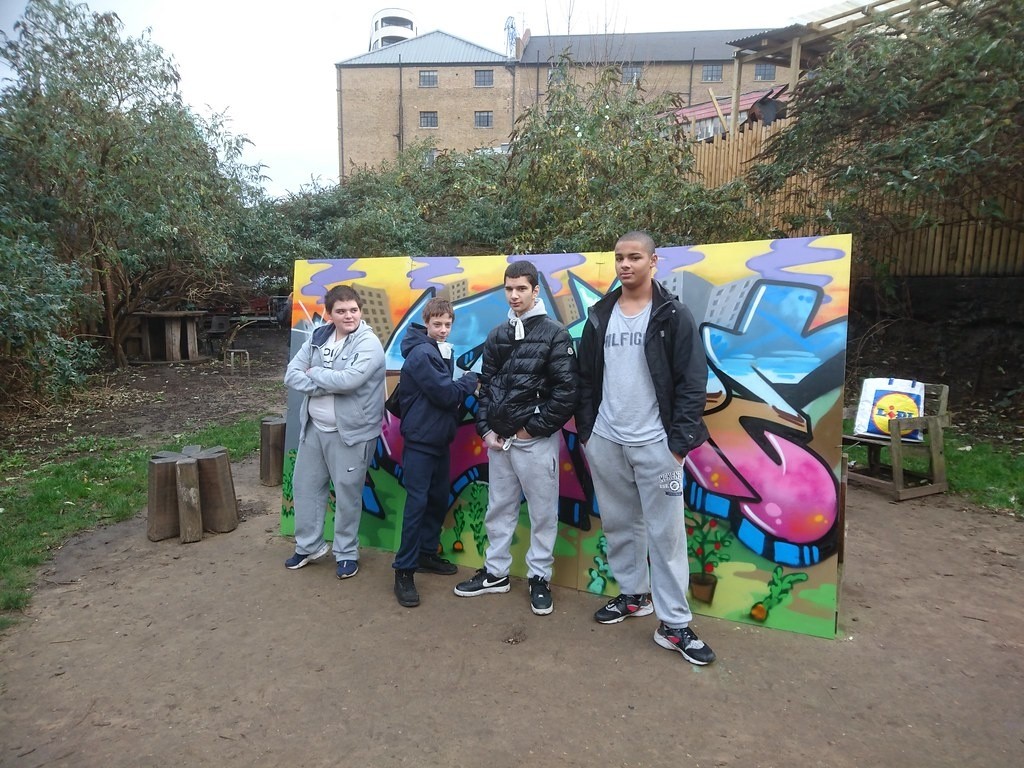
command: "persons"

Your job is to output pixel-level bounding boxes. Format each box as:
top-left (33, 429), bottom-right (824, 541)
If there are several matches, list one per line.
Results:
top-left (453, 260), bottom-right (578, 615)
top-left (282, 285), bottom-right (387, 580)
top-left (391, 299), bottom-right (458, 607)
top-left (576, 232), bottom-right (718, 667)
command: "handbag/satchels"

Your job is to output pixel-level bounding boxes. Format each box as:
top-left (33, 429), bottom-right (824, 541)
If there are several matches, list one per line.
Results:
top-left (854, 373), bottom-right (927, 442)
top-left (385, 382), bottom-right (401, 419)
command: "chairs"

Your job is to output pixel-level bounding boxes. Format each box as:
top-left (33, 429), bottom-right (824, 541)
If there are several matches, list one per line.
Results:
top-left (207, 315), bottom-right (231, 353)
top-left (842, 383), bottom-right (951, 502)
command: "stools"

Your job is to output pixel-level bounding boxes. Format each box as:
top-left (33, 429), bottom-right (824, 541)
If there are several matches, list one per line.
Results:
top-left (224, 349), bottom-right (251, 377)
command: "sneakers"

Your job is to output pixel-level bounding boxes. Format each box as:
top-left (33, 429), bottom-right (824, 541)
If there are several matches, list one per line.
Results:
top-left (336, 560), bottom-right (358, 579)
top-left (394, 569), bottom-right (420, 606)
top-left (594, 593), bottom-right (653, 623)
top-left (416, 553), bottom-right (457, 575)
top-left (454, 565), bottom-right (510, 596)
top-left (653, 620), bottom-right (717, 665)
top-left (528, 575), bottom-right (554, 615)
top-left (285, 542), bottom-right (329, 569)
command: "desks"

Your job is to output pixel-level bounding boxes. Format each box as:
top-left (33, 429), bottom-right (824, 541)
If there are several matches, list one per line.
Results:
top-left (133, 309), bottom-right (208, 361)
